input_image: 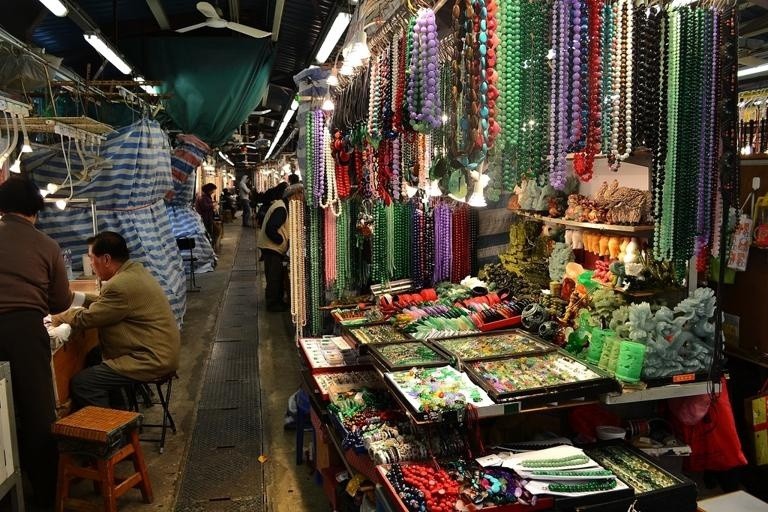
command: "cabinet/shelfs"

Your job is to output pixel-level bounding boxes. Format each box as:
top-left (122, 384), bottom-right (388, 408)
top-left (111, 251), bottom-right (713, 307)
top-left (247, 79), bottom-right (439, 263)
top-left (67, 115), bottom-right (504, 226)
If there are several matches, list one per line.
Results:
top-left (506, 148), bottom-right (662, 299)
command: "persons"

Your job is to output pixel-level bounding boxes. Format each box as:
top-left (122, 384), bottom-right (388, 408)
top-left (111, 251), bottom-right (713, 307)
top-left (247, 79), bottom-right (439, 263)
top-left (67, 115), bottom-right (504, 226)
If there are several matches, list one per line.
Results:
top-left (219, 174), bottom-right (300, 228)
top-left (0, 174), bottom-right (75, 512)
top-left (256, 183), bottom-right (306, 313)
top-left (43, 231), bottom-right (181, 409)
top-left (195, 182), bottom-right (221, 245)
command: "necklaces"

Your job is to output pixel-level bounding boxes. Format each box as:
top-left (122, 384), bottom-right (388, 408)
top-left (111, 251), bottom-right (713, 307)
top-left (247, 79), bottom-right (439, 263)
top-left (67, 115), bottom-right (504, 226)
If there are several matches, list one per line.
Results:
top-left (289, 199), bottom-right (475, 344)
top-left (385, 444), bottom-right (682, 511)
top-left (306, 1), bottom-right (741, 259)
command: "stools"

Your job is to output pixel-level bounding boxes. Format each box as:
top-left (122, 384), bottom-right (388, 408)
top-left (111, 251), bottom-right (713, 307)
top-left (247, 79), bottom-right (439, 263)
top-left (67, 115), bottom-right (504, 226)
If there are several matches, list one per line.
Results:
top-left (48, 405), bottom-right (154, 511)
top-left (115, 371), bottom-right (176, 453)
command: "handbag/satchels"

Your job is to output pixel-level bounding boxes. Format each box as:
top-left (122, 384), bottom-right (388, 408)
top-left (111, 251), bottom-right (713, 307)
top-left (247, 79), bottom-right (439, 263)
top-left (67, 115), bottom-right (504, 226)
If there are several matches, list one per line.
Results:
top-left (669, 378), bottom-right (748, 472)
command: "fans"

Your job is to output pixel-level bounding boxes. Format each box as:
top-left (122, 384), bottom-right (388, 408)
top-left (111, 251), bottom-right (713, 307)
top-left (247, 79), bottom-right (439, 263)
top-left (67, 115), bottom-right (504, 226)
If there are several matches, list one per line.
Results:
top-left (174, 1), bottom-right (273, 39)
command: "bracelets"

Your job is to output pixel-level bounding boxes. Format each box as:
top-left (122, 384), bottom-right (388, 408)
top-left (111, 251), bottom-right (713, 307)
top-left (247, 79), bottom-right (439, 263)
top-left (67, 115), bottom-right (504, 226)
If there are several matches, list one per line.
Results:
top-left (328, 387), bottom-right (468, 465)
top-left (383, 287), bottom-right (533, 333)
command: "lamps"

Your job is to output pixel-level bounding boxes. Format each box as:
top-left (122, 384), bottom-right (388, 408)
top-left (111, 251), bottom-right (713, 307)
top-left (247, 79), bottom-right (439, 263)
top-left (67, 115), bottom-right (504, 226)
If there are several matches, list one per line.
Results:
top-left (83, 29), bottom-right (133, 75)
top-left (0, 99), bottom-right (108, 211)
top-left (319, 0), bottom-right (386, 111)
